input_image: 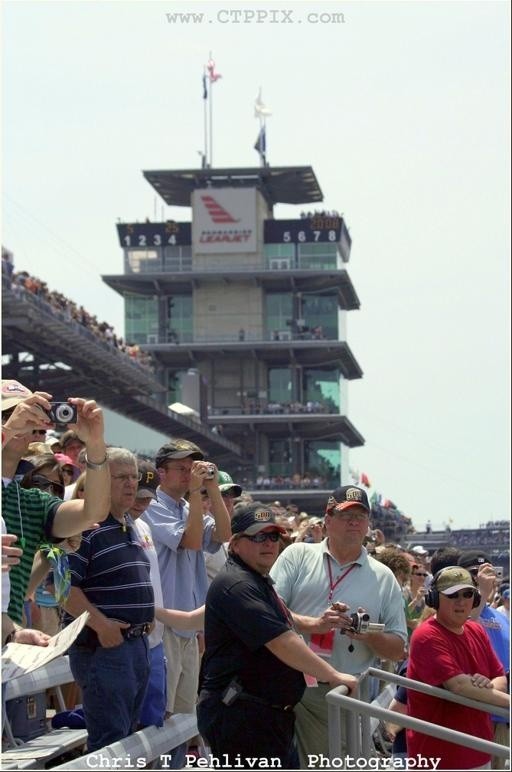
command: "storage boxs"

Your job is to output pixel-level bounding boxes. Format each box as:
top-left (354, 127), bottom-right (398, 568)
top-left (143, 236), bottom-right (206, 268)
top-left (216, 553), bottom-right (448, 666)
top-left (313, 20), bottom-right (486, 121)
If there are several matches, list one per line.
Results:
top-left (5, 689), bottom-right (50, 743)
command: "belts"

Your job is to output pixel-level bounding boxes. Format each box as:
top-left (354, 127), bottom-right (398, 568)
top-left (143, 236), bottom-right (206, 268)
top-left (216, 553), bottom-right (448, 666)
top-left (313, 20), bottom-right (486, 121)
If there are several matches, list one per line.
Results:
top-left (77, 622), bottom-right (155, 644)
top-left (222, 694), bottom-right (296, 719)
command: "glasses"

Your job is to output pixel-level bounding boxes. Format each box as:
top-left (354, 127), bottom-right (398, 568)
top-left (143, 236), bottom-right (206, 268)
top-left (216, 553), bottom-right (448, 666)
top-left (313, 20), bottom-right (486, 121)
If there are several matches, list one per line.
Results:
top-left (242, 532), bottom-right (280, 543)
top-left (111, 472), bottom-right (143, 482)
top-left (33, 474), bottom-right (64, 496)
top-left (442, 589), bottom-right (473, 600)
top-left (415, 572), bottom-right (427, 578)
top-left (31, 429), bottom-right (46, 436)
top-left (59, 466), bottom-right (74, 476)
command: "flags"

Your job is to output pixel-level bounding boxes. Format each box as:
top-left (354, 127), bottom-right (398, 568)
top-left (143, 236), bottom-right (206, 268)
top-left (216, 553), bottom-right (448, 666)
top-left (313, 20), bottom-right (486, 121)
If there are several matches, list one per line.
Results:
top-left (252, 124), bottom-right (266, 157)
top-left (200, 58), bottom-right (223, 98)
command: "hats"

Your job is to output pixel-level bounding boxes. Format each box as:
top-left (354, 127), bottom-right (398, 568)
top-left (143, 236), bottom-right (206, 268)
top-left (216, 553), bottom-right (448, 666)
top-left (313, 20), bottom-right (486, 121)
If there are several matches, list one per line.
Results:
top-left (325, 485), bottom-right (370, 517)
top-left (215, 470), bottom-right (242, 499)
top-left (307, 517), bottom-right (322, 526)
top-left (434, 566), bottom-right (476, 595)
top-left (1, 379), bottom-right (33, 411)
top-left (134, 460), bottom-right (160, 503)
top-left (410, 545), bottom-right (429, 557)
top-left (397, 552), bottom-right (419, 569)
top-left (155, 440), bottom-right (204, 466)
top-left (52, 452), bottom-right (82, 487)
top-left (458, 550), bottom-right (492, 571)
top-left (46, 706), bottom-right (90, 731)
top-left (231, 501), bottom-right (286, 537)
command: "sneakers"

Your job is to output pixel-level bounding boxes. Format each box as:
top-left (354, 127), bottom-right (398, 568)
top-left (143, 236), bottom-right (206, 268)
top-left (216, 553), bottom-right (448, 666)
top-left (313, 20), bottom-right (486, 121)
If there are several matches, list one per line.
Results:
top-left (372, 717), bottom-right (396, 761)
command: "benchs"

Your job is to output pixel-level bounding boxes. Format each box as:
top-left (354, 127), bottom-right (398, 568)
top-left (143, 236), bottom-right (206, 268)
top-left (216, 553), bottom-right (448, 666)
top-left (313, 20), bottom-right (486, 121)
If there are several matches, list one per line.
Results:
top-left (1, 654), bottom-right (89, 770)
top-left (49, 707), bottom-right (216, 770)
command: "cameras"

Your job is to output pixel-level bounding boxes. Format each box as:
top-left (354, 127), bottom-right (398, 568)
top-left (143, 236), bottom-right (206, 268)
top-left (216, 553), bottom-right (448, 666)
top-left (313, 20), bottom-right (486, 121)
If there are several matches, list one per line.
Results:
top-left (201, 465), bottom-right (214, 480)
top-left (351, 613), bottom-right (385, 634)
top-left (38, 402), bottom-right (78, 424)
